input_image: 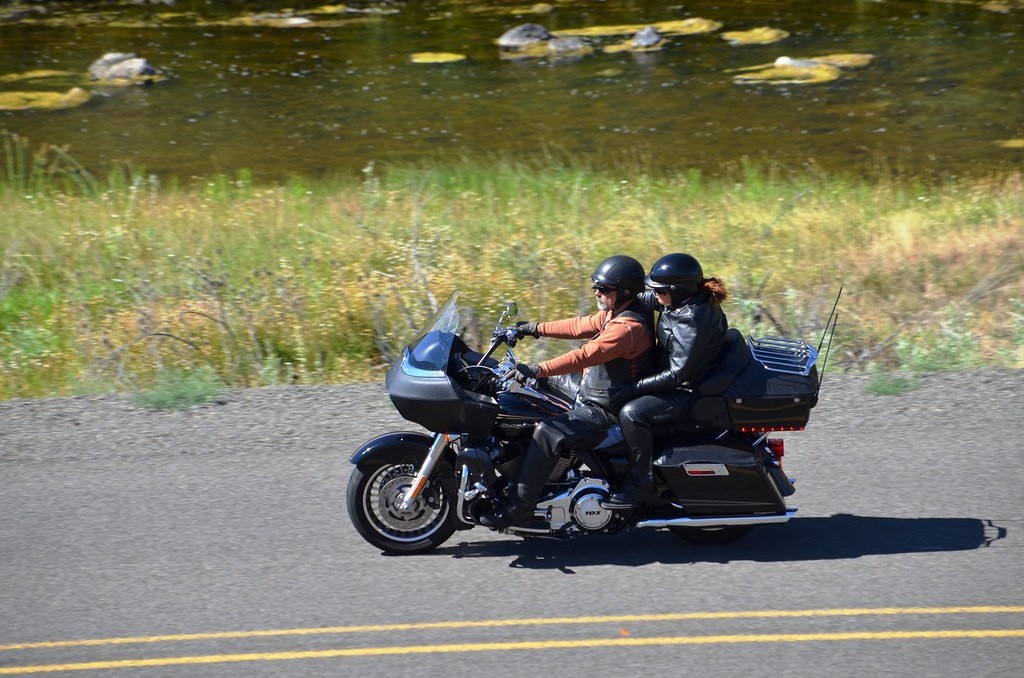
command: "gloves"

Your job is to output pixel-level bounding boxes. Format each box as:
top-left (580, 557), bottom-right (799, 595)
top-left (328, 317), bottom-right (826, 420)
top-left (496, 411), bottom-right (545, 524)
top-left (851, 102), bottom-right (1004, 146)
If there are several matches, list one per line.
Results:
top-left (509, 320), bottom-right (541, 341)
top-left (514, 362), bottom-right (543, 384)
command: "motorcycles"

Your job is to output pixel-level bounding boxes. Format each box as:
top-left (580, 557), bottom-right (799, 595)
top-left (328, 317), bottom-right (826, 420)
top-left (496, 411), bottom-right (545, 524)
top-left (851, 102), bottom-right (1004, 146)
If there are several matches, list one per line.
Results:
top-left (345, 281), bottom-right (819, 554)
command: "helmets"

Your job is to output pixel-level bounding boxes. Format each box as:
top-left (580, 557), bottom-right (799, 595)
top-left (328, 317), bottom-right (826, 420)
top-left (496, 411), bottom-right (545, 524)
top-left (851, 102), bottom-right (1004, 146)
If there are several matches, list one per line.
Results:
top-left (591, 255), bottom-right (646, 292)
top-left (645, 253), bottom-right (704, 293)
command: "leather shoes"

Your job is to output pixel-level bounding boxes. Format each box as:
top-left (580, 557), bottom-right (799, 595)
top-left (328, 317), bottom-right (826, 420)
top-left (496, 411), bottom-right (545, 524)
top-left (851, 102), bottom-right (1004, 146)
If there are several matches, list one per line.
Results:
top-left (611, 489), bottom-right (651, 504)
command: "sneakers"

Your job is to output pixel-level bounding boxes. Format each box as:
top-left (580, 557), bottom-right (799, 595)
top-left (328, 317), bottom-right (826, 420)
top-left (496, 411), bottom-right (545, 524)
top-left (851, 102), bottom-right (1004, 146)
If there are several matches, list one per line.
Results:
top-left (479, 494), bottom-right (535, 528)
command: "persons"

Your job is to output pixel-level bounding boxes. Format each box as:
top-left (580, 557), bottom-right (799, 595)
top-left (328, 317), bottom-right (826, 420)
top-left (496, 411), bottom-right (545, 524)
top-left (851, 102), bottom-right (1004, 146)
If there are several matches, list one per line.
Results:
top-left (610, 254), bottom-right (728, 503)
top-left (468, 255), bottom-right (656, 527)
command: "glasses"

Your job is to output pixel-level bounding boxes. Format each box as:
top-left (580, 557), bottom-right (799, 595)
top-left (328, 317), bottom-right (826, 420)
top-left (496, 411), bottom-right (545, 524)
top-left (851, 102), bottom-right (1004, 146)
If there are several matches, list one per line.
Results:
top-left (593, 285), bottom-right (619, 295)
top-left (652, 288), bottom-right (667, 296)
